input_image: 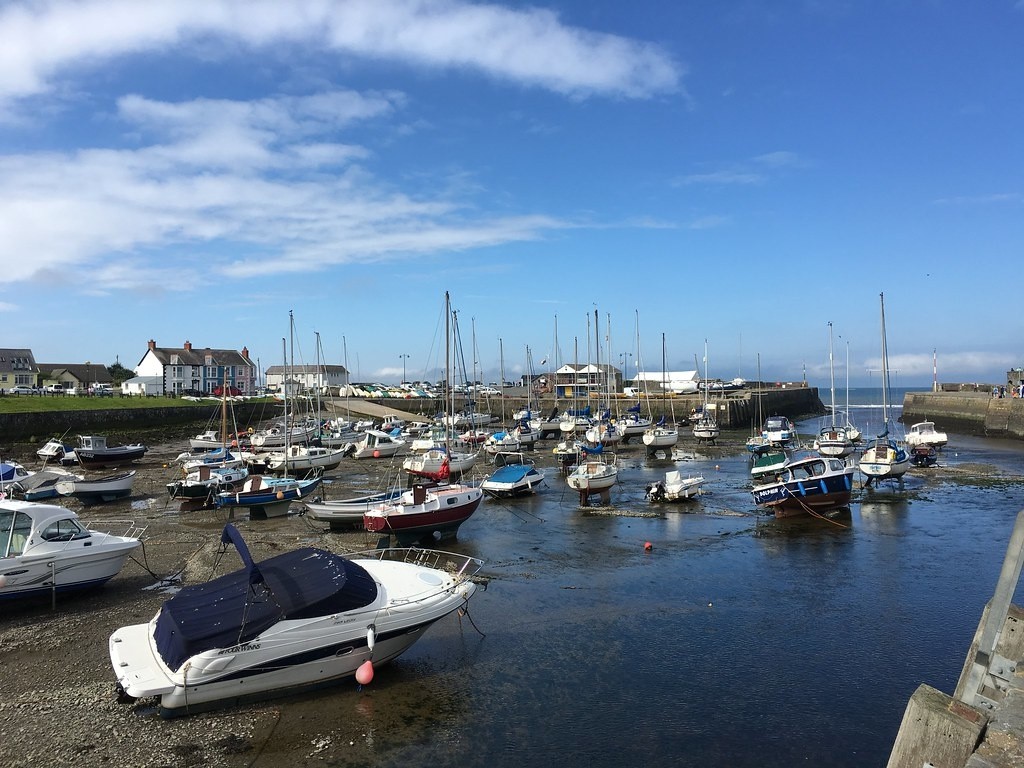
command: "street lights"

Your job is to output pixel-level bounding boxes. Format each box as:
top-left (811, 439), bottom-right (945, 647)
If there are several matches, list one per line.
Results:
top-left (620, 352), bottom-right (633, 387)
top-left (399, 353), bottom-right (410, 389)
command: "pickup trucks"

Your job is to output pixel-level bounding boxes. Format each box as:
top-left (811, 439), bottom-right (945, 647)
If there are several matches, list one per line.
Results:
top-left (480, 387), bottom-right (502, 396)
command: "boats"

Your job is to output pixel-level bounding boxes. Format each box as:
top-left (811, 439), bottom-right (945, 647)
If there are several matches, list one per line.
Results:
top-left (54, 469), bottom-right (137, 505)
top-left (909, 443), bottom-right (938, 467)
top-left (751, 456), bottom-right (855, 518)
top-left (0, 499), bottom-right (150, 612)
top-left (481, 451), bottom-right (546, 500)
top-left (304, 488), bottom-right (413, 522)
top-left (762, 416), bottom-right (795, 448)
top-left (663, 470), bottom-right (705, 500)
top-left (37, 426), bottom-right (71, 464)
top-left (108, 522), bottom-right (486, 722)
top-left (73, 435), bottom-right (146, 470)
top-left (0, 461), bottom-right (85, 502)
top-left (904, 419), bottom-right (948, 447)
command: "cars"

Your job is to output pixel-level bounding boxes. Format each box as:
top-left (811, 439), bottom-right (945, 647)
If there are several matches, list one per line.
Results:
top-left (96, 384), bottom-right (113, 394)
top-left (413, 381), bottom-right (512, 386)
top-left (9, 385), bottom-right (40, 395)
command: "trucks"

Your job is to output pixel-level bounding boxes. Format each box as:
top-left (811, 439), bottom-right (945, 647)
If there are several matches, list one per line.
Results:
top-left (400, 385), bottom-right (415, 391)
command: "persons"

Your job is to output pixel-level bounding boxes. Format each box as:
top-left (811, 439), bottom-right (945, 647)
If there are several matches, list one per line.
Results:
top-left (88, 385), bottom-right (96, 397)
top-left (31, 383), bottom-right (36, 389)
top-left (973, 383), bottom-right (978, 392)
top-left (992, 384), bottom-right (1024, 399)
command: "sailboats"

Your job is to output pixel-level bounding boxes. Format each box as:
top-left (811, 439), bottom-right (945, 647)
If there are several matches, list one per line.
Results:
top-left (362, 290), bottom-right (484, 548)
top-left (165, 303), bottom-right (654, 521)
top-left (858, 292), bottom-right (910, 478)
top-left (692, 338), bottom-right (720, 440)
top-left (812, 321), bottom-right (856, 458)
top-left (840, 341), bottom-right (862, 442)
top-left (746, 353), bottom-right (773, 456)
top-left (643, 333), bottom-right (679, 450)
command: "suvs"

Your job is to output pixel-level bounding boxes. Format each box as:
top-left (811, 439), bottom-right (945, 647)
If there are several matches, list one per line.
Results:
top-left (46, 384), bottom-right (63, 394)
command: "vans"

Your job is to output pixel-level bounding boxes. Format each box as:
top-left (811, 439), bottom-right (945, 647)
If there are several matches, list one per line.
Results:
top-left (624, 387), bottom-right (643, 396)
